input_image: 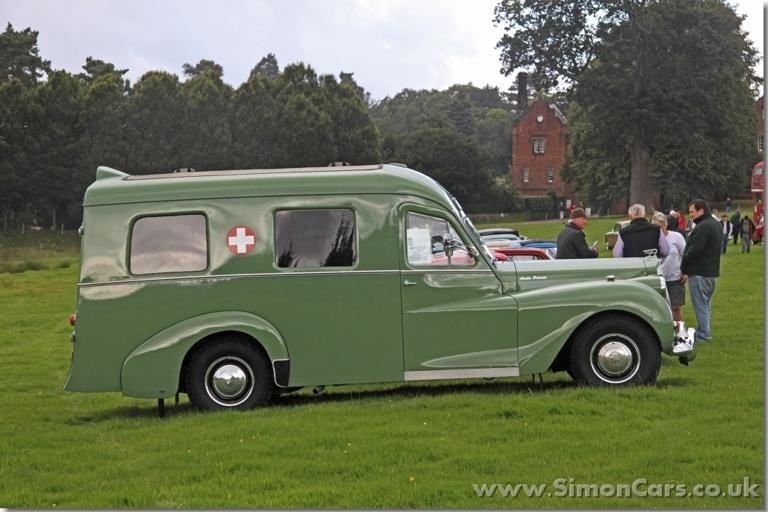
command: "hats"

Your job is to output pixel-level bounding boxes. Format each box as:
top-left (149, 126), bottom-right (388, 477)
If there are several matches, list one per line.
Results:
top-left (570, 208), bottom-right (585, 218)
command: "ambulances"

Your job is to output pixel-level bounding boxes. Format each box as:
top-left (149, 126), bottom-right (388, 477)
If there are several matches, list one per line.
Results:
top-left (61, 158), bottom-right (697, 414)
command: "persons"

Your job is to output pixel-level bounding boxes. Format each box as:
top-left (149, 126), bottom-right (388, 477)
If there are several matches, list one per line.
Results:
top-left (613, 203), bottom-right (670, 258)
top-left (559, 201), bottom-right (566, 220)
top-left (567, 203), bottom-right (577, 215)
top-left (554, 207), bottom-right (600, 259)
top-left (680, 199), bottom-right (723, 344)
top-left (651, 212), bottom-right (688, 321)
top-left (664, 207), bottom-right (764, 255)
top-left (724, 197), bottom-right (733, 213)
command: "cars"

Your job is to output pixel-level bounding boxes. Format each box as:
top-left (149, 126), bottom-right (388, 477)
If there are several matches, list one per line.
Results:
top-left (683, 215), bottom-right (697, 236)
top-left (424, 218), bottom-right (560, 262)
top-left (604, 219), bottom-right (633, 251)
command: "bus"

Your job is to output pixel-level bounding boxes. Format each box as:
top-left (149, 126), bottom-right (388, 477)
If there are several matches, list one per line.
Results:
top-left (750, 160), bottom-right (765, 241)
